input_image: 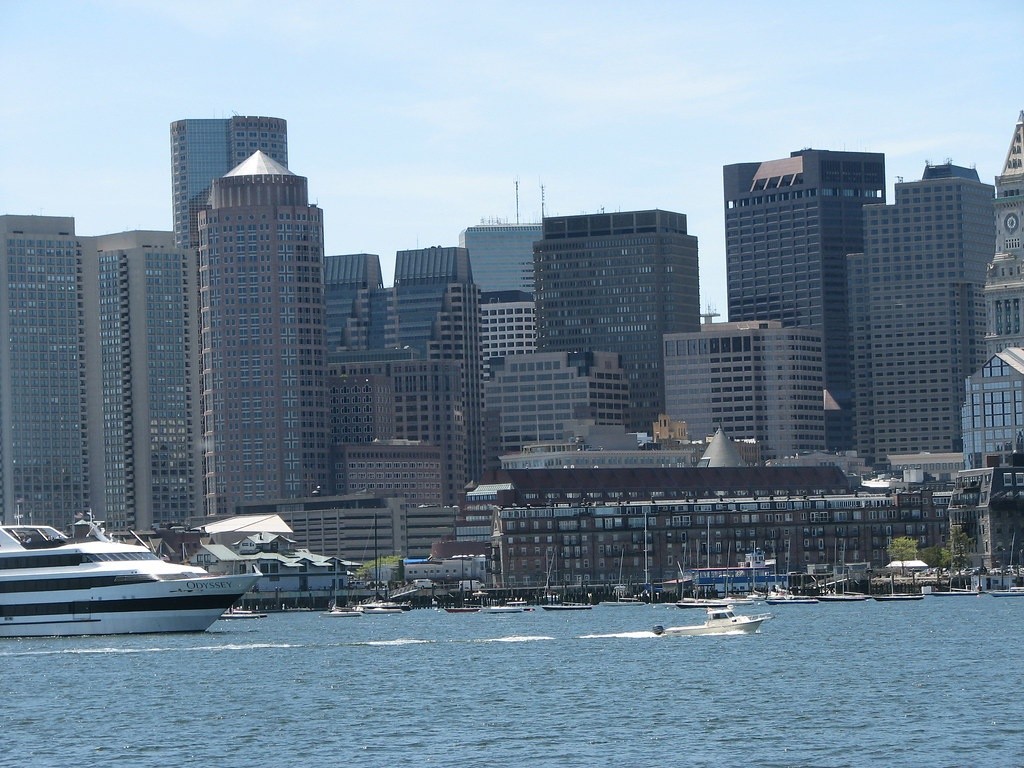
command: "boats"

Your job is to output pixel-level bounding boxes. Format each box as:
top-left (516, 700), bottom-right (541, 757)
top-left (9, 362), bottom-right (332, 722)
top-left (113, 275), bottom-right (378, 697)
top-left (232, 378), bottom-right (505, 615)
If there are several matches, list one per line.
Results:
top-left (219, 606), bottom-right (267, 620)
top-left (490, 602), bottom-right (535, 613)
top-left (542, 602), bottom-right (592, 610)
top-left (874, 594), bottom-right (925, 601)
top-left (989, 587), bottom-right (1024, 597)
top-left (323, 607), bottom-right (362, 617)
top-left (599, 598), bottom-right (646, 606)
top-left (0, 526), bottom-right (263, 637)
top-left (353, 599), bottom-right (403, 614)
top-left (653, 608), bottom-right (775, 634)
top-left (765, 591), bottom-right (872, 605)
top-left (685, 547), bottom-right (789, 593)
top-left (676, 584), bottom-right (754, 608)
top-left (932, 588), bottom-right (979, 596)
top-left (444, 604), bottom-right (483, 614)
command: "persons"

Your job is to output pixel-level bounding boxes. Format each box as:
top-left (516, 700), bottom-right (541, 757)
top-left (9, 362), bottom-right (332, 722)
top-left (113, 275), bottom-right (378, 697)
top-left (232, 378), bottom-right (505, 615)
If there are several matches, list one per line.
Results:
top-left (44, 533), bottom-right (53, 541)
top-left (20, 533), bottom-right (31, 542)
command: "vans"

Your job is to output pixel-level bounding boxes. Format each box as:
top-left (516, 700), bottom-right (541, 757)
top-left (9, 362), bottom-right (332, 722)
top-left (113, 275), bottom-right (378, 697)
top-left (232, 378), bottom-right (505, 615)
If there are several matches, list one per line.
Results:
top-left (459, 580), bottom-right (486, 590)
top-left (350, 581), bottom-right (366, 590)
top-left (412, 579), bottom-right (437, 590)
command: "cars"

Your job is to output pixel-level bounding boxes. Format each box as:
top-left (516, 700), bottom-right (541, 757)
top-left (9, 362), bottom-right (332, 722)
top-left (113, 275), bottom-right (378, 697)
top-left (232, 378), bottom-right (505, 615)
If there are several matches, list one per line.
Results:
top-left (908, 564), bottom-right (1024, 576)
